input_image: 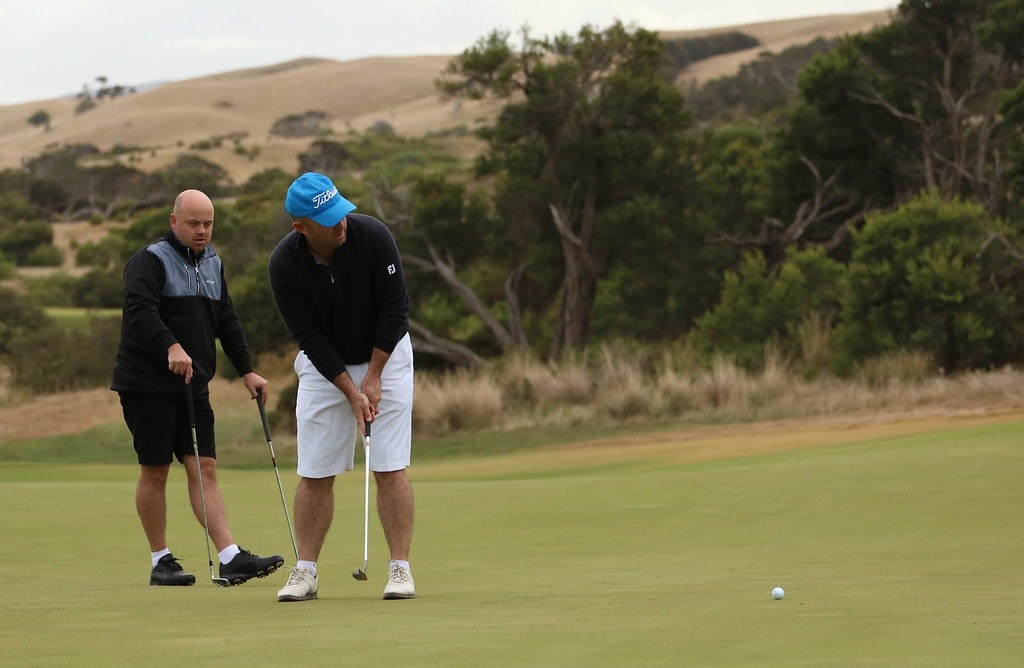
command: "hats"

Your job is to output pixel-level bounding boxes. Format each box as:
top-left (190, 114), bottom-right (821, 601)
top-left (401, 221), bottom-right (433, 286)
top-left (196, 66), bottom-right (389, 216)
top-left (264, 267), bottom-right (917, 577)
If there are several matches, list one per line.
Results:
top-left (284, 172), bottom-right (357, 229)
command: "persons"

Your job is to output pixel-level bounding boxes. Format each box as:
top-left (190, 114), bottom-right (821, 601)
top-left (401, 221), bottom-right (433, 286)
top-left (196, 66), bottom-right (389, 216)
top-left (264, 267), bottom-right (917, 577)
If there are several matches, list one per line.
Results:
top-left (275, 172), bottom-right (414, 599)
top-left (109, 189), bottom-right (283, 587)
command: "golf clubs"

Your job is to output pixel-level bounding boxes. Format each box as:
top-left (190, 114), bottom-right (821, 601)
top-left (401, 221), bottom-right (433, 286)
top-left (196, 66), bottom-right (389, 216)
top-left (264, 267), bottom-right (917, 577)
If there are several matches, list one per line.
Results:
top-left (255, 388), bottom-right (299, 562)
top-left (351, 418), bottom-right (371, 581)
top-left (183, 375), bottom-right (230, 587)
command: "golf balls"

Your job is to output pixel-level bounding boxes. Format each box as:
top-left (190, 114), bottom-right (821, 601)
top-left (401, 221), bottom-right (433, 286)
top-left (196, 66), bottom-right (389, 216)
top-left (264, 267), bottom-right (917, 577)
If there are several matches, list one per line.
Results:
top-left (771, 587), bottom-right (785, 600)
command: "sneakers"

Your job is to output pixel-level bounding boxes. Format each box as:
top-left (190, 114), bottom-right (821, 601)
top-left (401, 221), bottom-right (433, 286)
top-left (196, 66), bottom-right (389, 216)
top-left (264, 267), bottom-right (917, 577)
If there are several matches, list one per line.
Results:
top-left (382, 565), bottom-right (415, 598)
top-left (276, 565), bottom-right (319, 601)
top-left (219, 546), bottom-right (284, 586)
top-left (148, 553), bottom-right (196, 586)
